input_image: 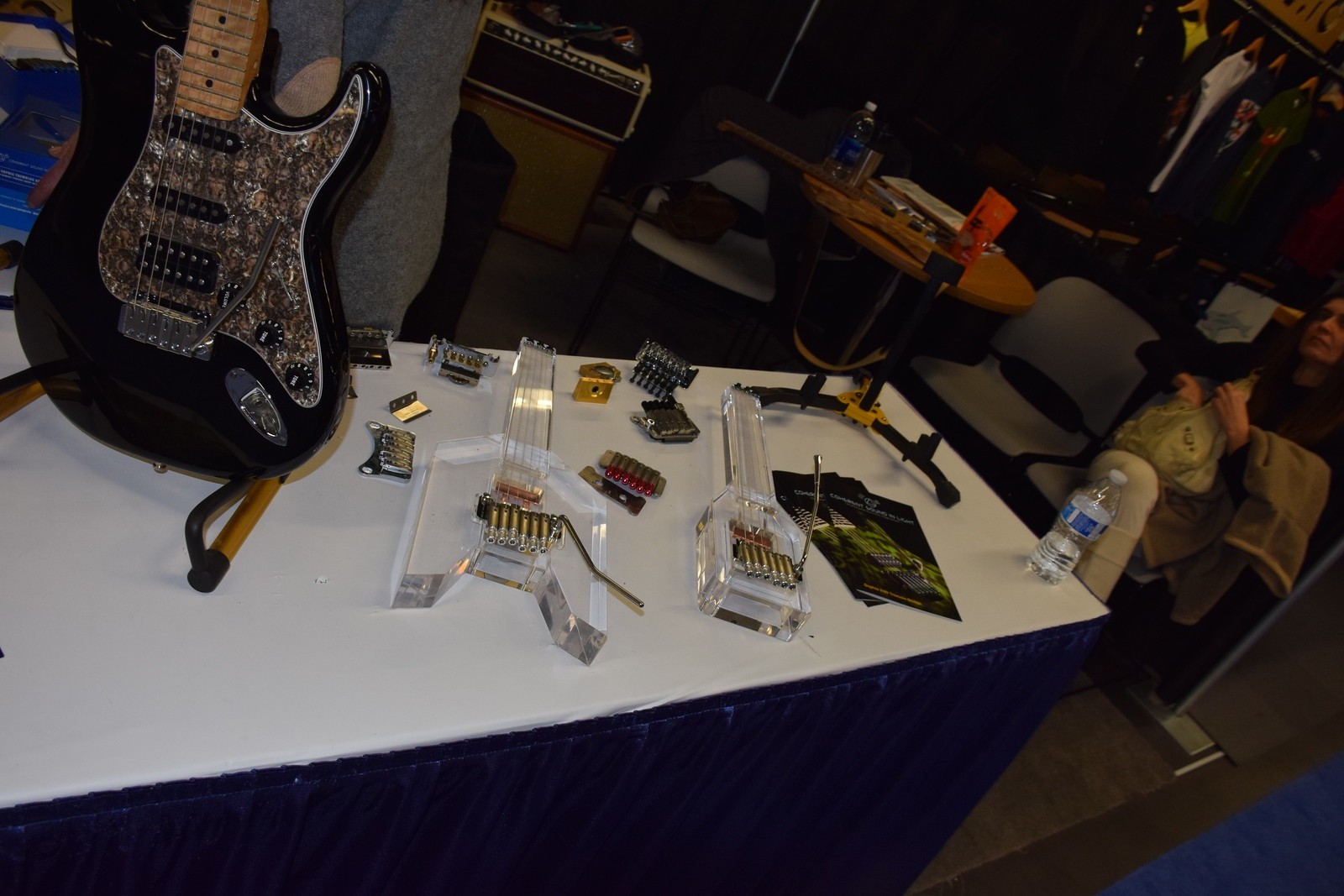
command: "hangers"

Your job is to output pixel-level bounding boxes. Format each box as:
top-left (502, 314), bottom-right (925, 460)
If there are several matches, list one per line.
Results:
top-left (1177, 0), bottom-right (1344, 112)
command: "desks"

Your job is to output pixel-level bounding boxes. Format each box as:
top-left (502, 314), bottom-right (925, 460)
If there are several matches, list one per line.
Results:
top-left (1, 267), bottom-right (1114, 896)
top-left (791, 170), bottom-right (1039, 381)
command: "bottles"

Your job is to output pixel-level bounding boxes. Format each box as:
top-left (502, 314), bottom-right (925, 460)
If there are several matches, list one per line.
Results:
top-left (824, 102), bottom-right (877, 179)
top-left (1028, 468), bottom-right (1128, 584)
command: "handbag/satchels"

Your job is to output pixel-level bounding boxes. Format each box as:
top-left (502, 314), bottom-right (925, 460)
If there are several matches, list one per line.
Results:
top-left (1114, 375), bottom-right (1255, 497)
top-left (624, 178), bottom-right (740, 245)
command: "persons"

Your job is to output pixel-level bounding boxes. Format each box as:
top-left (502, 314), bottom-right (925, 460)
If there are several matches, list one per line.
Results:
top-left (1075, 290), bottom-right (1344, 605)
top-left (28, 0), bottom-right (482, 341)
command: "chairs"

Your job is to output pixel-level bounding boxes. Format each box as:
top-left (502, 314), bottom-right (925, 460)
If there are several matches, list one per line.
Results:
top-left (908, 276), bottom-right (1251, 582)
top-left (629, 85), bottom-right (818, 365)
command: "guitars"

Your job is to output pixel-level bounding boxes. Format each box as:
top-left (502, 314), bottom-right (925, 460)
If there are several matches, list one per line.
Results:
top-left (388, 334), bottom-right (609, 667)
top-left (695, 383), bottom-right (824, 642)
top-left (11, 0), bottom-right (390, 481)
